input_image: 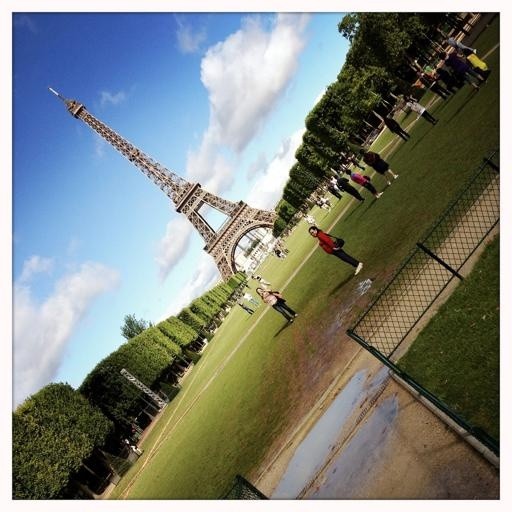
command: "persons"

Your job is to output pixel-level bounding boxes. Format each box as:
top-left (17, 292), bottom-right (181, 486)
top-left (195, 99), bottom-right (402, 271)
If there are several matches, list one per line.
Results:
top-left (235, 38), bottom-right (492, 324)
top-left (124, 438), bottom-right (143, 457)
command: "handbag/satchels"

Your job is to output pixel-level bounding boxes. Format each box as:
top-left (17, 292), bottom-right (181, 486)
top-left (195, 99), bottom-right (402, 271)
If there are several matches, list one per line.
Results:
top-left (334, 238), bottom-right (344, 248)
top-left (363, 176), bottom-right (370, 181)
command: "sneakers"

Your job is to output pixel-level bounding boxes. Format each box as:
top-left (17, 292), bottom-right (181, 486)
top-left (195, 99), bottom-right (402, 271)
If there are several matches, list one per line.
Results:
top-left (355, 262), bottom-right (363, 276)
top-left (387, 181), bottom-right (391, 185)
top-left (295, 314), bottom-right (299, 317)
top-left (376, 192), bottom-right (383, 199)
top-left (433, 119), bottom-right (439, 124)
top-left (290, 319), bottom-right (293, 323)
top-left (393, 174), bottom-right (398, 179)
